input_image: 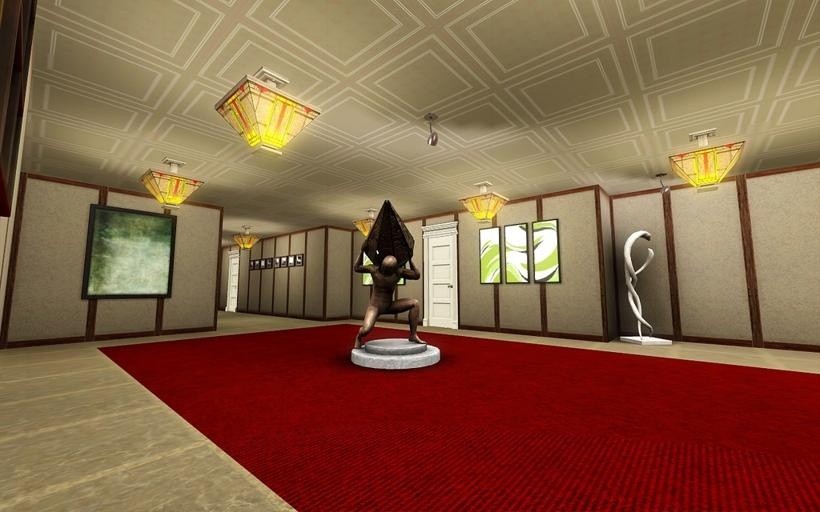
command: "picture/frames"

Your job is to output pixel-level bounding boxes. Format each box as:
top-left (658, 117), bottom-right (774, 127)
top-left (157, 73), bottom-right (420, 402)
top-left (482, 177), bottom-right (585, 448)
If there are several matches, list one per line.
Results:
top-left (362, 251), bottom-right (406, 286)
top-left (250, 254), bottom-right (303, 270)
top-left (532, 219), bottom-right (561, 283)
top-left (504, 223), bottom-right (530, 284)
top-left (479, 226), bottom-right (502, 284)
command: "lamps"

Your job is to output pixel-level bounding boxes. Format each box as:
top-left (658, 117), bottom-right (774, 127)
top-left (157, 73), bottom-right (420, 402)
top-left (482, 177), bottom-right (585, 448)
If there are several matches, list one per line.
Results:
top-left (138, 157), bottom-right (205, 211)
top-left (233, 225), bottom-right (261, 250)
top-left (352, 208), bottom-right (378, 239)
top-left (458, 181), bottom-right (510, 224)
top-left (668, 128), bottom-right (745, 194)
top-left (214, 68), bottom-right (321, 160)
